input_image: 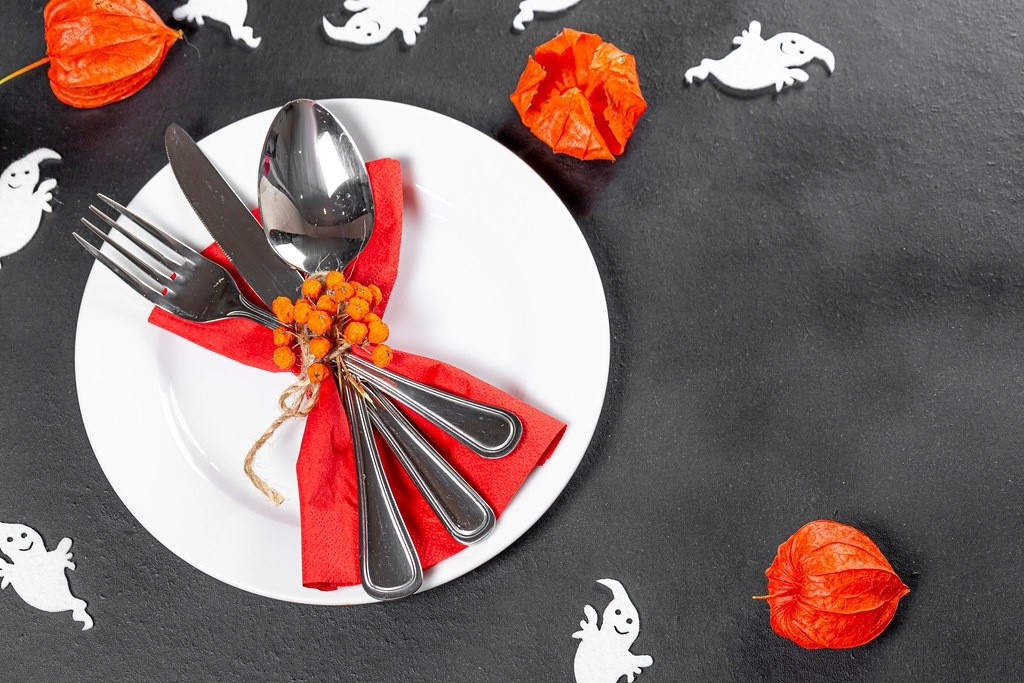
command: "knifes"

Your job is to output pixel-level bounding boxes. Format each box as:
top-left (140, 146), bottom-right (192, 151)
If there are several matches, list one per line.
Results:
top-left (163, 121), bottom-right (498, 546)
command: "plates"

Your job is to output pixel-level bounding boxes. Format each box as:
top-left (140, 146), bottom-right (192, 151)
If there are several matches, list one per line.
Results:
top-left (73, 96), bottom-right (610, 607)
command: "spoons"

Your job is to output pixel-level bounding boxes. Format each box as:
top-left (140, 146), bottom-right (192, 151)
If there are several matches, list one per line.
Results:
top-left (256, 99), bottom-right (424, 603)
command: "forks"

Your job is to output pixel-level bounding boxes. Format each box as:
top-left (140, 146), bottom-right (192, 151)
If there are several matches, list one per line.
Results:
top-left (72, 191), bottom-right (523, 458)
